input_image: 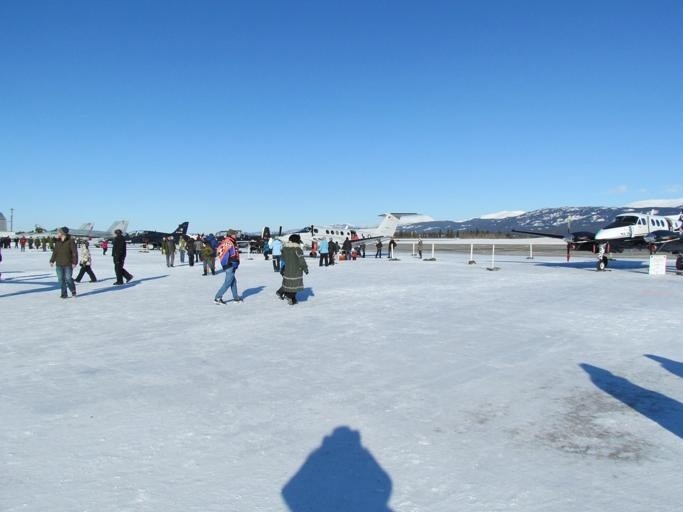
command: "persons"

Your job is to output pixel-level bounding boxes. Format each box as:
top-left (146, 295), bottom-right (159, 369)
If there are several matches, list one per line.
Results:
top-left (48, 227), bottom-right (76, 299)
top-left (162, 234), bottom-right (220, 276)
top-left (416, 238), bottom-right (423, 259)
top-left (318, 236), bottom-right (366, 267)
top-left (211, 227), bottom-right (243, 305)
top-left (72, 238), bottom-right (97, 283)
top-left (386, 236), bottom-right (397, 258)
top-left (274, 233), bottom-right (308, 306)
top-left (111, 229), bottom-right (134, 286)
top-left (102, 239), bottom-right (107, 255)
top-left (0, 235), bottom-right (58, 253)
top-left (248, 235), bottom-right (283, 273)
top-left (375, 238), bottom-right (383, 258)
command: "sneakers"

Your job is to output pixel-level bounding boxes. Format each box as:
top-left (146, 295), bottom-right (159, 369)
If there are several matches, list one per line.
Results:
top-left (113, 282), bottom-right (124, 285)
top-left (276, 289), bottom-right (285, 300)
top-left (126, 275), bottom-right (133, 282)
top-left (214, 298), bottom-right (226, 305)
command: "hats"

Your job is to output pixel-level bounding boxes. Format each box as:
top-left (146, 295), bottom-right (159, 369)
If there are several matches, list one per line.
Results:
top-left (59, 227), bottom-right (70, 234)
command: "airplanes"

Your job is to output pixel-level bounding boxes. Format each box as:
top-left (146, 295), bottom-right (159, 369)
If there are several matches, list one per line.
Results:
top-left (511, 212), bottom-right (683, 272)
top-left (211, 225), bottom-right (290, 253)
top-left (271, 212), bottom-right (421, 255)
top-left (18, 217), bottom-right (131, 245)
top-left (123, 221), bottom-right (195, 249)
